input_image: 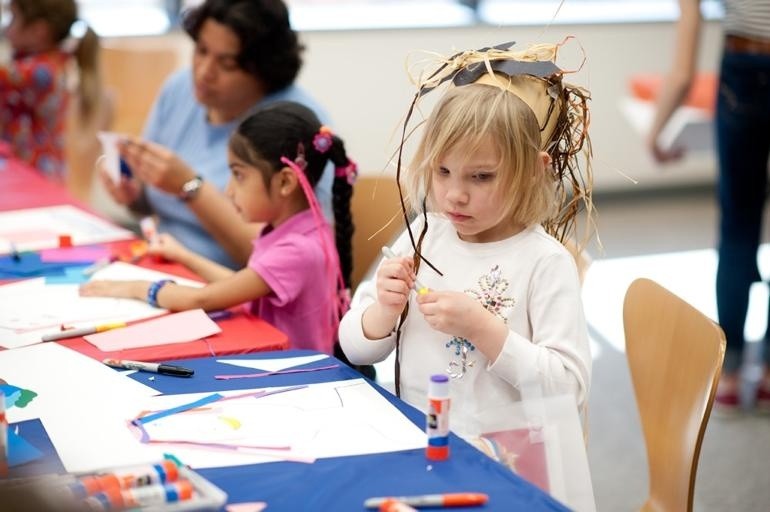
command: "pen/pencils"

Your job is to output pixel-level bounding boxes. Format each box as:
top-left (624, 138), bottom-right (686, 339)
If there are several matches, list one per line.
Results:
top-left (85, 255), bottom-right (118, 275)
top-left (103, 358), bottom-right (194, 377)
top-left (364, 493), bottom-right (489, 512)
top-left (42, 320), bottom-right (127, 341)
top-left (382, 246), bottom-right (430, 294)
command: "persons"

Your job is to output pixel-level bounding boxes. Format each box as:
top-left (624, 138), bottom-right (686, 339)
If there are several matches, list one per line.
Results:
top-left (645, 0), bottom-right (770, 404)
top-left (337, 42), bottom-right (596, 512)
top-left (97, 0), bottom-right (338, 271)
top-left (0, 0), bottom-right (98, 186)
top-left (80, 104), bottom-right (358, 354)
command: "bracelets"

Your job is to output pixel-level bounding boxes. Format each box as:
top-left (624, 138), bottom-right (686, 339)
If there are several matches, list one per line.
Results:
top-left (180, 176), bottom-right (203, 202)
top-left (148, 280), bottom-right (175, 307)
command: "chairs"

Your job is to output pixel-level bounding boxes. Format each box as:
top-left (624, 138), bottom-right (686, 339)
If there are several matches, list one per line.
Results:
top-left (349, 176), bottom-right (412, 297)
top-left (623, 278), bottom-right (726, 512)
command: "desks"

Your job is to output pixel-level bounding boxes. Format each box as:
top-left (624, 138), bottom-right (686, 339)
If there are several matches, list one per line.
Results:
top-left (0, 155), bottom-right (292, 384)
top-left (0, 349), bottom-right (572, 512)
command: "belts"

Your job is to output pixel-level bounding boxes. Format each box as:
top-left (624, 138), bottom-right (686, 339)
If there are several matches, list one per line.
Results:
top-left (725, 35), bottom-right (770, 54)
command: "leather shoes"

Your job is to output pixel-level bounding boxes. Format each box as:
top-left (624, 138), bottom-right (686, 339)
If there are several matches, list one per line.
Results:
top-left (716, 394), bottom-right (740, 406)
top-left (756, 388), bottom-right (770, 401)
